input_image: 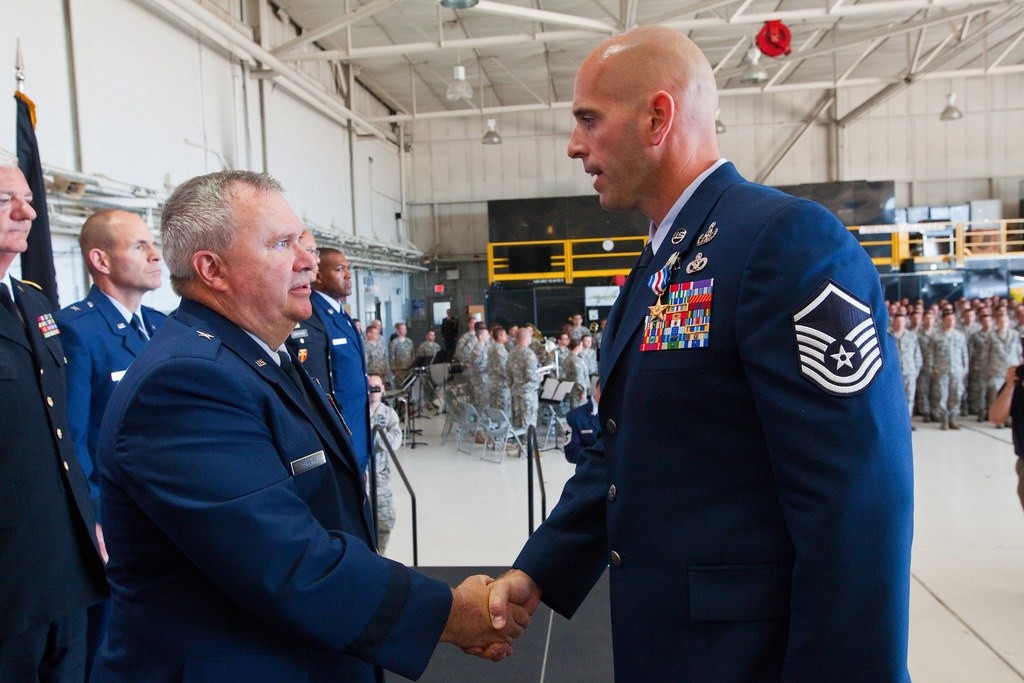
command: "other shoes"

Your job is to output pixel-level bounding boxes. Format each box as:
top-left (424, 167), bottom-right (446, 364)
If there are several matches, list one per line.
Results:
top-left (977, 410), bottom-right (989, 422)
top-left (940, 417), bottom-right (959, 430)
top-left (996, 416), bottom-right (1013, 428)
top-left (912, 426), bottom-right (917, 432)
top-left (469, 428), bottom-right (492, 444)
top-left (922, 414), bottom-right (942, 423)
top-left (960, 400), bottom-right (979, 417)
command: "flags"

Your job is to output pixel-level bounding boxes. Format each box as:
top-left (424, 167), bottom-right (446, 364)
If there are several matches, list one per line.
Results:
top-left (14, 91), bottom-right (61, 314)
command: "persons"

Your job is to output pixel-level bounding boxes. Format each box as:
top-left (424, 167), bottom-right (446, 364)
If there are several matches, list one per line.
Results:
top-left (459, 317), bottom-right (544, 458)
top-left (462, 27), bottom-right (915, 683)
top-left (352, 318), bottom-right (439, 393)
top-left (281, 247), bottom-right (369, 481)
top-left (52, 211), bottom-right (170, 496)
top-left (363, 375), bottom-right (402, 555)
top-left (884, 294), bottom-right (1024, 434)
top-left (88, 170), bottom-right (529, 683)
top-left (545, 315), bottom-right (609, 449)
top-left (990, 335), bottom-right (1024, 509)
top-left (0, 160), bottom-right (108, 683)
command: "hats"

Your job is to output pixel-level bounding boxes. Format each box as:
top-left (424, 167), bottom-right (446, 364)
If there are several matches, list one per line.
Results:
top-left (941, 308), bottom-right (954, 319)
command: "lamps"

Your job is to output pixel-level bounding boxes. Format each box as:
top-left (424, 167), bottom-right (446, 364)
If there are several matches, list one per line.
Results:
top-left (715, 109), bottom-right (726, 134)
top-left (42, 173), bottom-right (90, 202)
top-left (446, 52), bottom-right (474, 101)
top-left (483, 118), bottom-right (501, 145)
top-left (741, 24), bottom-right (768, 84)
top-left (940, 78), bottom-right (963, 120)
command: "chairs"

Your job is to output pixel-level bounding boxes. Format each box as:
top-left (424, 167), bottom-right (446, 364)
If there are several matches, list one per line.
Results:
top-left (441, 374), bottom-right (527, 465)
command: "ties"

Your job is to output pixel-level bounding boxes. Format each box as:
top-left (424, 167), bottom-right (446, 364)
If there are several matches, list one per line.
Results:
top-left (0, 282), bottom-right (28, 340)
top-left (130, 312), bottom-right (151, 345)
top-left (340, 304), bottom-right (348, 323)
top-left (620, 241), bottom-right (654, 319)
top-left (277, 349), bottom-right (309, 407)
